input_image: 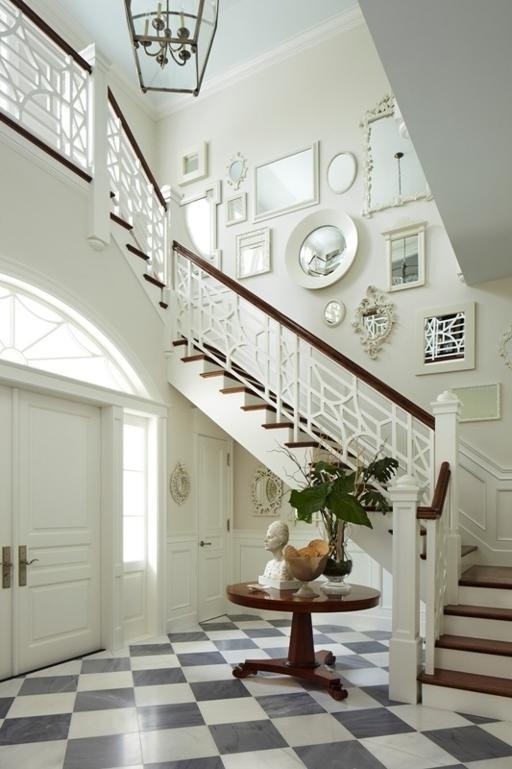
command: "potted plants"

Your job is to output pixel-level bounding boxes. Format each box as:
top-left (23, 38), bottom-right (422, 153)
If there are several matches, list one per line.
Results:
top-left (267, 426), bottom-right (398, 596)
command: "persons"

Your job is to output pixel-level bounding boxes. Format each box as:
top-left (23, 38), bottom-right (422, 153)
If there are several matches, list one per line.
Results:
top-left (258, 521), bottom-right (302, 590)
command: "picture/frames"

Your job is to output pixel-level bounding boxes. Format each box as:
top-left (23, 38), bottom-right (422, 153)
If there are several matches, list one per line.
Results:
top-left (252, 140), bottom-right (320, 224)
top-left (286, 209), bottom-right (358, 289)
top-left (380, 221), bottom-right (426, 293)
top-left (236, 227), bottom-right (271, 280)
top-left (177, 142), bottom-right (208, 187)
top-left (226, 193), bottom-right (247, 227)
top-left (352, 286), bottom-right (395, 360)
top-left (452, 382), bottom-right (500, 423)
top-left (414, 302), bottom-right (476, 376)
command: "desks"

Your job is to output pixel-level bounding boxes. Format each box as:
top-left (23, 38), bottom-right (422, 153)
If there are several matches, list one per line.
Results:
top-left (227, 581), bottom-right (381, 700)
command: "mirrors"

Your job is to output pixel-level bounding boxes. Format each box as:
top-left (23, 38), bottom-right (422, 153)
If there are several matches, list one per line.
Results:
top-left (170, 462), bottom-right (190, 504)
top-left (248, 468), bottom-right (282, 516)
top-left (322, 299), bottom-right (345, 328)
top-left (225, 152), bottom-right (248, 190)
top-left (327, 152), bottom-right (358, 194)
top-left (357, 93), bottom-right (434, 218)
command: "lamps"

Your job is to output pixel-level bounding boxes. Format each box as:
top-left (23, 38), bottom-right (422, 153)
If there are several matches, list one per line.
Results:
top-left (124, 0), bottom-right (219, 97)
